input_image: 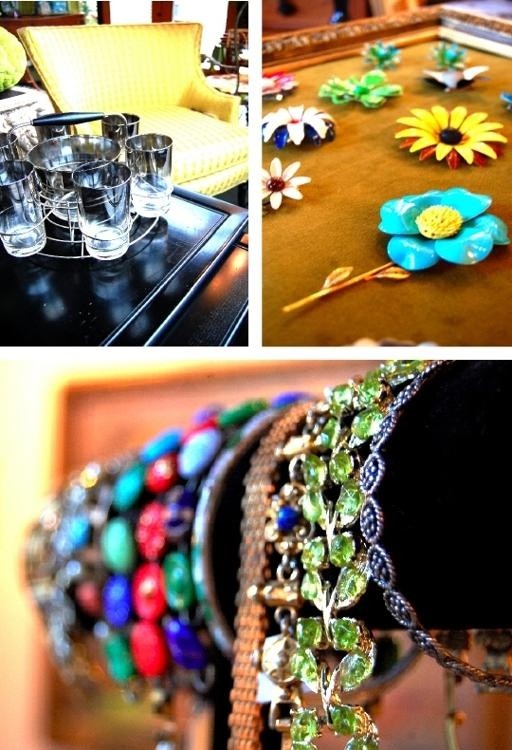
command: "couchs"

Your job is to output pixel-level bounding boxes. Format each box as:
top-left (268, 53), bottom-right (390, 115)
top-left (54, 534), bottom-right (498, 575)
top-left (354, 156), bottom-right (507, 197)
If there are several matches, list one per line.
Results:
top-left (19, 22), bottom-right (247, 193)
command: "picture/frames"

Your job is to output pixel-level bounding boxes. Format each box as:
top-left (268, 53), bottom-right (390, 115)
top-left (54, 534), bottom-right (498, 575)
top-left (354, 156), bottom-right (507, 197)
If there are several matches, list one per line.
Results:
top-left (263, 4), bottom-right (512, 346)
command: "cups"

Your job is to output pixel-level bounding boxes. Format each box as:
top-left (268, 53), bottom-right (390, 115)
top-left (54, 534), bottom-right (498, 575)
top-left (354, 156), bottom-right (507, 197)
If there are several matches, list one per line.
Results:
top-left (71, 161), bottom-right (133, 260)
top-left (1, 157), bottom-right (47, 259)
top-left (101, 110), bottom-right (141, 148)
top-left (0, 132), bottom-right (19, 162)
top-left (123, 131), bottom-right (175, 218)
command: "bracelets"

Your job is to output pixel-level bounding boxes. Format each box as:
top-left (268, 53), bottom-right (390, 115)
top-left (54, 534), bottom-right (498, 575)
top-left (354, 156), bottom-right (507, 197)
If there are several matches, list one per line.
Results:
top-left (25, 356), bottom-right (512, 750)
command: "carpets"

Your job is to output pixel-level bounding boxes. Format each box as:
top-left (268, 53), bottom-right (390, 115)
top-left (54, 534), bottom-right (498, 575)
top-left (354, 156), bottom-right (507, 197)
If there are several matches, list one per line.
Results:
top-left (0, 186), bottom-right (249, 347)
